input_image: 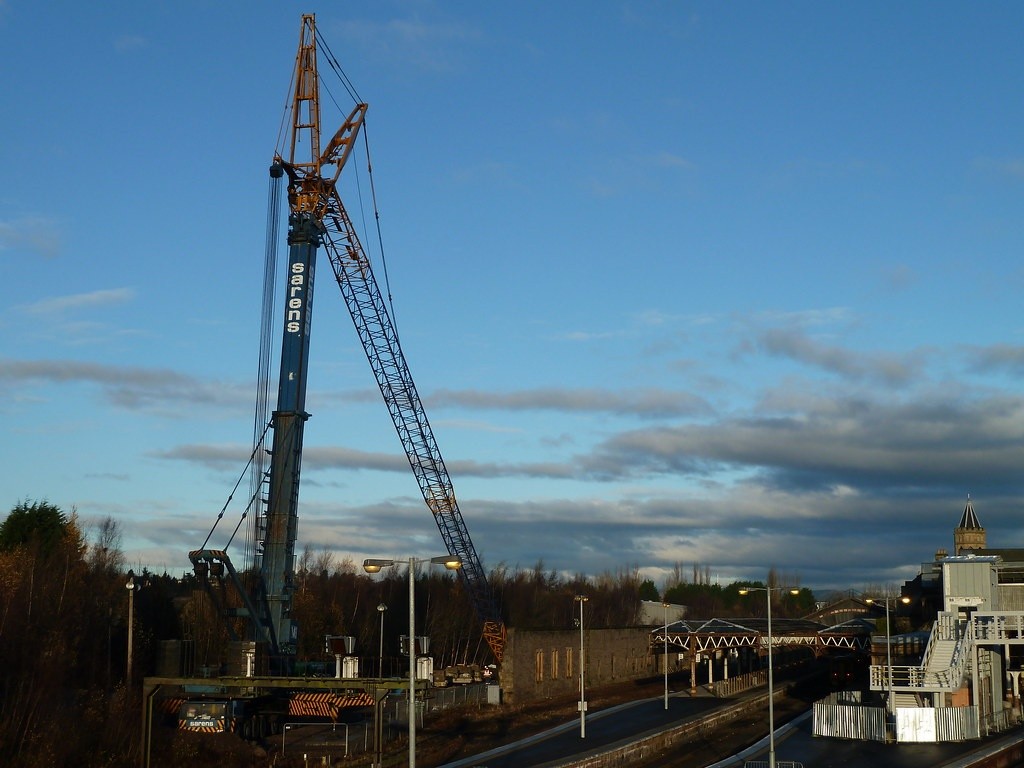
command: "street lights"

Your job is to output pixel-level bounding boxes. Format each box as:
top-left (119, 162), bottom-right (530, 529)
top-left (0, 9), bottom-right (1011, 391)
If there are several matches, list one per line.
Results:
top-left (575, 594), bottom-right (589, 739)
top-left (363, 555), bottom-right (463, 768)
top-left (865, 596), bottom-right (911, 713)
top-left (739, 583), bottom-right (800, 768)
top-left (662, 602), bottom-right (671, 709)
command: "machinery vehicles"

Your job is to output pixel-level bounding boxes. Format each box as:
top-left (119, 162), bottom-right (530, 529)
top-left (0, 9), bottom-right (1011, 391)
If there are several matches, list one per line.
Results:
top-left (120, 12), bottom-right (509, 768)
